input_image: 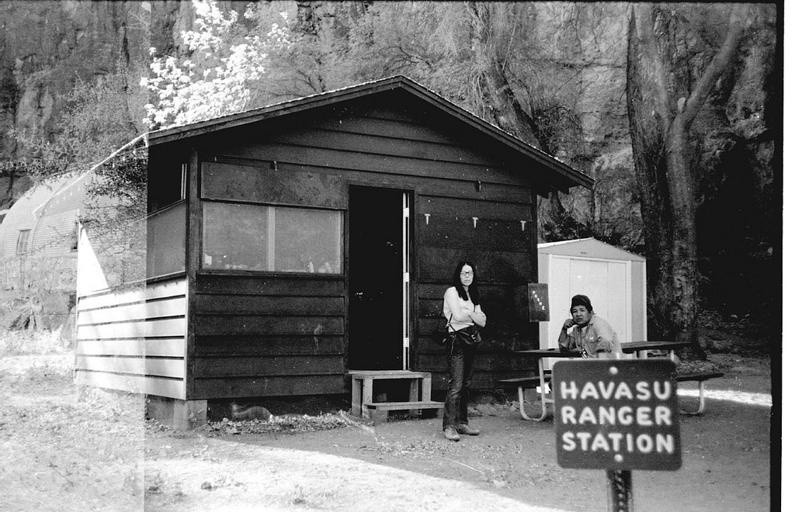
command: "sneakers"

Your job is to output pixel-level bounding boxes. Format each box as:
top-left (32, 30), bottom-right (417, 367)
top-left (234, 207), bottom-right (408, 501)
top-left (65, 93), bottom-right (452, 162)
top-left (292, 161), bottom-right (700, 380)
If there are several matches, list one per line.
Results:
top-left (444, 424), bottom-right (460, 442)
top-left (456, 422), bottom-right (481, 436)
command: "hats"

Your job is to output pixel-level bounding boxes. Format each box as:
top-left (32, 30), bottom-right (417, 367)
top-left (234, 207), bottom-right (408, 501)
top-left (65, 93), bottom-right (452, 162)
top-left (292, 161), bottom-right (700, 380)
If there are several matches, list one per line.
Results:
top-left (570, 294), bottom-right (593, 315)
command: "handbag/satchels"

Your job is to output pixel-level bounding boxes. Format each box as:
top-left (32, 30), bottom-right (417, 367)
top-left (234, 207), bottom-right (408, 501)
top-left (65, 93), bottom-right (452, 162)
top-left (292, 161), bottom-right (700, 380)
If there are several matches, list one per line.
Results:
top-left (456, 324), bottom-right (483, 352)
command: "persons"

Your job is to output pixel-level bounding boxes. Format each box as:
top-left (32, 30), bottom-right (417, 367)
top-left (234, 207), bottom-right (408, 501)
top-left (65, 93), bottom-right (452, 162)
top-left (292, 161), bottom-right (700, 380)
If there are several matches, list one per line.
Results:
top-left (441, 260), bottom-right (488, 441)
top-left (558, 294), bottom-right (624, 359)
